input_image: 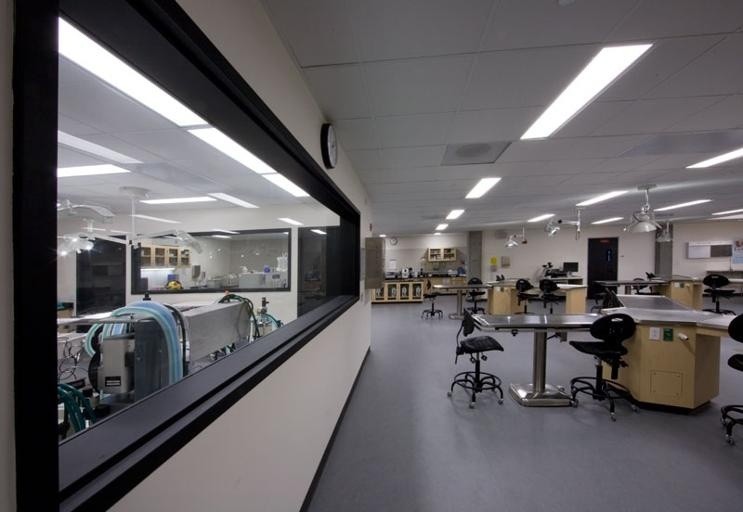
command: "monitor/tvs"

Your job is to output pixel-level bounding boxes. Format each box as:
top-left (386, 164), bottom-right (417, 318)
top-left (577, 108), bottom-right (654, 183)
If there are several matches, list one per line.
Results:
top-left (563, 262), bottom-right (578, 272)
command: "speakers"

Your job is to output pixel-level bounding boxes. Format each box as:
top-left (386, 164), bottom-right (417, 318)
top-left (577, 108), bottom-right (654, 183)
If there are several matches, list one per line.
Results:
top-left (191, 265), bottom-right (200, 278)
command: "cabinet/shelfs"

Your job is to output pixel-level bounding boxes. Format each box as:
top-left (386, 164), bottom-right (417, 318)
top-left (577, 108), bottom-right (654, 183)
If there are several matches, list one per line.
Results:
top-left (370, 282), bottom-right (424, 303)
top-left (428, 248), bottom-right (457, 262)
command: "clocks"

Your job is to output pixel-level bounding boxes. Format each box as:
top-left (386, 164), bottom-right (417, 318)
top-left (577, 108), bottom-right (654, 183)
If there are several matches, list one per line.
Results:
top-left (389, 238), bottom-right (398, 245)
top-left (321, 122), bottom-right (337, 167)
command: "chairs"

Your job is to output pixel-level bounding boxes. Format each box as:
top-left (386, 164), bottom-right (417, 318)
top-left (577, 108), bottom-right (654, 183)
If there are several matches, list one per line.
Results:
top-left (423, 280), bottom-right (443, 320)
top-left (448, 310), bottom-right (504, 407)
top-left (722, 313), bottom-right (742, 445)
top-left (464, 277), bottom-right (486, 314)
top-left (489, 274), bottom-right (742, 314)
top-left (571, 313), bottom-right (637, 420)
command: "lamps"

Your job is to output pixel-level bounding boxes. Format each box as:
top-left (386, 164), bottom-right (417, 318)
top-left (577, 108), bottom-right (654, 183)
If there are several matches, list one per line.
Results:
top-left (628, 190), bottom-right (657, 234)
top-left (544, 219), bottom-right (562, 240)
top-left (501, 233), bottom-right (518, 247)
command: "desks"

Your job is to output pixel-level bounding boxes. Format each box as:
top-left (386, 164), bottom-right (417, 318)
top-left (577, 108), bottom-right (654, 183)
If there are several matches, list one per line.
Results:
top-left (434, 284), bottom-right (493, 320)
top-left (472, 312), bottom-right (616, 407)
top-left (602, 308), bottom-right (732, 410)
